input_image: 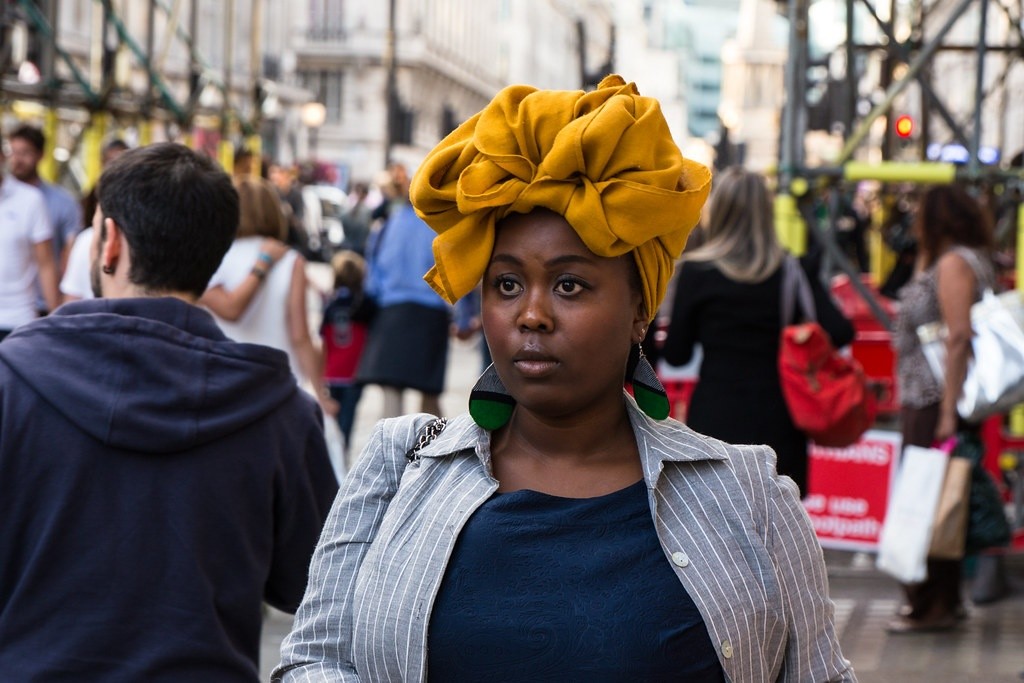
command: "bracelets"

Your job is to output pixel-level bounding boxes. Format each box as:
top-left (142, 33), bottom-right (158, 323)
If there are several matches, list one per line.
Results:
top-left (250, 266), bottom-right (266, 280)
top-left (257, 252), bottom-right (273, 267)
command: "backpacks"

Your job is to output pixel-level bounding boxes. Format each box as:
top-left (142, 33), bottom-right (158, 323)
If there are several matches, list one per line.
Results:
top-left (318, 283), bottom-right (381, 386)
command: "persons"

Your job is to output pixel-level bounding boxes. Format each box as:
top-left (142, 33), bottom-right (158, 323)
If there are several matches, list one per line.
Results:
top-left (0, 143), bottom-right (341, 682)
top-left (0, 122), bottom-right (492, 486)
top-left (270, 73), bottom-right (858, 683)
top-left (638, 150), bottom-right (1024, 634)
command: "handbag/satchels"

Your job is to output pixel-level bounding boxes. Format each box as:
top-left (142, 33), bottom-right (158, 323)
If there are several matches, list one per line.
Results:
top-left (779, 251), bottom-right (880, 449)
top-left (798, 428), bottom-right (977, 585)
top-left (915, 249), bottom-right (1024, 424)
